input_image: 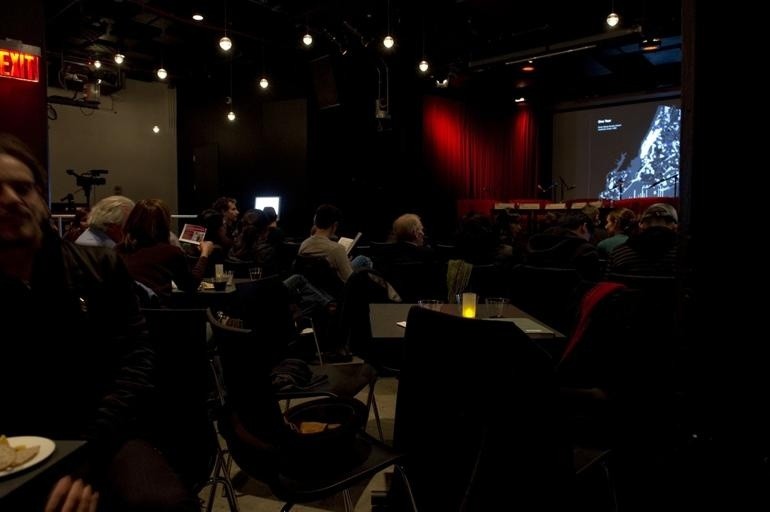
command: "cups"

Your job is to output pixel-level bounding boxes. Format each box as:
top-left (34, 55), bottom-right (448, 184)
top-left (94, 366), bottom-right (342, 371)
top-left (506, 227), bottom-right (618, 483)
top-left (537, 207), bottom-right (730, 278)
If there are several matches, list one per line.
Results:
top-left (455, 292), bottom-right (479, 319)
top-left (418, 300), bottom-right (443, 311)
top-left (485, 298), bottom-right (505, 318)
top-left (212, 271), bottom-right (233, 291)
top-left (249, 268), bottom-right (262, 281)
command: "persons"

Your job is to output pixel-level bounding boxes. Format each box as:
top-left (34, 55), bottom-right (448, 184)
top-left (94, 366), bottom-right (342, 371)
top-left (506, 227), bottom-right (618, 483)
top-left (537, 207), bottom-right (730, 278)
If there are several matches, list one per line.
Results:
top-left (1, 133), bottom-right (678, 512)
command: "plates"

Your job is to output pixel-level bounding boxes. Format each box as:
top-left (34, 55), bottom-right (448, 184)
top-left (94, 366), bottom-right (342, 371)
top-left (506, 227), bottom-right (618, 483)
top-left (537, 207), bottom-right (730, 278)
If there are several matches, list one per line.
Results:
top-left (203, 282), bottom-right (214, 289)
top-left (0, 436), bottom-right (56, 477)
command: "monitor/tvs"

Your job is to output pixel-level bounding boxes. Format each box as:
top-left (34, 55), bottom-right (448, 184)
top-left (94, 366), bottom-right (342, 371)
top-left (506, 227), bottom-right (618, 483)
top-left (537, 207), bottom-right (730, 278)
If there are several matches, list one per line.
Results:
top-left (253, 195), bottom-right (282, 223)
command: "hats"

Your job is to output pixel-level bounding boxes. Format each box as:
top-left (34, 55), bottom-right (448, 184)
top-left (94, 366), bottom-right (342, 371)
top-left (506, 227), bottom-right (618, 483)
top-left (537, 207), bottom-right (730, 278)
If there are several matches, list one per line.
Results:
top-left (641, 202), bottom-right (679, 223)
